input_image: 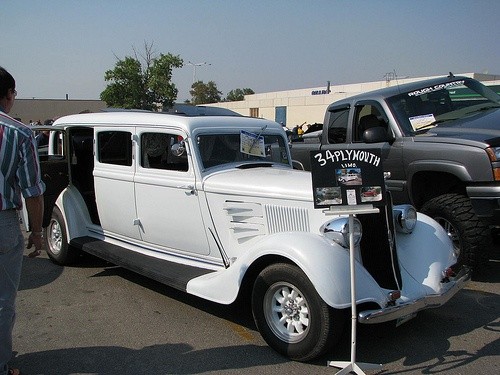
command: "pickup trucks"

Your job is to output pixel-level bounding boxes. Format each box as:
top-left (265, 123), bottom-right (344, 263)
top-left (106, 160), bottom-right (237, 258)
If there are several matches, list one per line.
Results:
top-left (255, 70), bottom-right (500, 275)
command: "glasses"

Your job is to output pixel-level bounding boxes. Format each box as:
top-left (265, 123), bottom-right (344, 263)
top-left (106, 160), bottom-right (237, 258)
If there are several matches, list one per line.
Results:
top-left (10, 89), bottom-right (17, 97)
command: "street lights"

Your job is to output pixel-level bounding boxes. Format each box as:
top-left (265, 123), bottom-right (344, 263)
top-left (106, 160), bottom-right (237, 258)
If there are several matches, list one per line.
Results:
top-left (184, 61), bottom-right (212, 83)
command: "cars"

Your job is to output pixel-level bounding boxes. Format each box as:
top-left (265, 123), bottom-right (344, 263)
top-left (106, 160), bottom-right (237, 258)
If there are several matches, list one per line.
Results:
top-left (20, 103), bottom-right (472, 365)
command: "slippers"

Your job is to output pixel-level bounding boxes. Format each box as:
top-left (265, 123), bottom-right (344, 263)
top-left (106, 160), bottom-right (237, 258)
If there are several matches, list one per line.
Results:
top-left (7, 368), bottom-right (21, 375)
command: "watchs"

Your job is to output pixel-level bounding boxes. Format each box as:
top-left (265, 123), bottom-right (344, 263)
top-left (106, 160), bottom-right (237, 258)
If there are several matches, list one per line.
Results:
top-left (32, 227), bottom-right (44, 238)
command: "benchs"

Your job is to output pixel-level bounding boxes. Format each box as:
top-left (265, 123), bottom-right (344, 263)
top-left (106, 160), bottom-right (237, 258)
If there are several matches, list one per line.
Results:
top-left (72, 132), bottom-right (163, 176)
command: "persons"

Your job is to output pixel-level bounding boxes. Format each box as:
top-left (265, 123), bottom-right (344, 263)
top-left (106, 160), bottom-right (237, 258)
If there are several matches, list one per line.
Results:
top-left (29, 119), bottom-right (54, 146)
top-left (292, 122), bottom-right (311, 135)
top-left (0, 67), bottom-right (46, 375)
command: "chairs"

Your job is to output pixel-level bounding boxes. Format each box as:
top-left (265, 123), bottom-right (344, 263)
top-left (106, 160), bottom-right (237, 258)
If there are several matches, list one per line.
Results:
top-left (358, 115), bottom-right (390, 139)
top-left (420, 97), bottom-right (451, 114)
